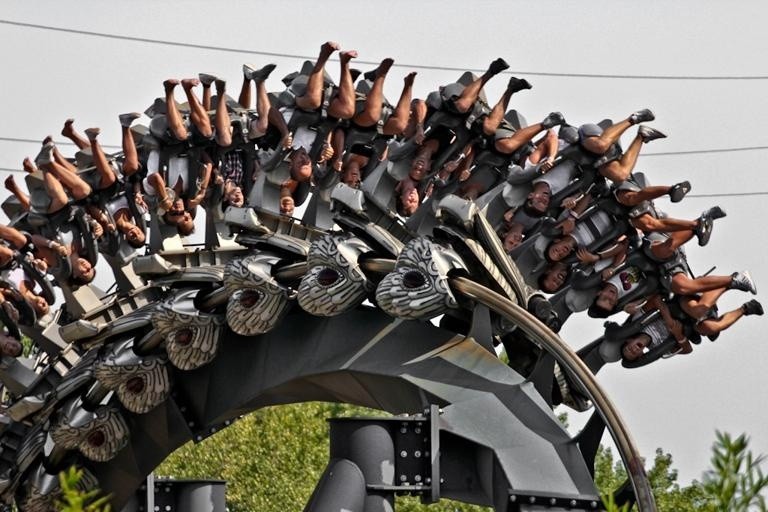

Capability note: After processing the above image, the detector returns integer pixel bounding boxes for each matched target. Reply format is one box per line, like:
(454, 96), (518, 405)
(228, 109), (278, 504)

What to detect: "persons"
(0, 41), (765, 360)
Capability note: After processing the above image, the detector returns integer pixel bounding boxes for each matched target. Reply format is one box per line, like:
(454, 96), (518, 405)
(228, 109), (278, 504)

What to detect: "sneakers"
(35, 140), (54, 169)
(668, 180), (692, 203)
(119, 110), (140, 129)
(542, 112), (566, 128)
(199, 71), (215, 87)
(630, 107), (655, 124)
(702, 206), (726, 220)
(507, 75), (533, 93)
(742, 298), (764, 316)
(490, 58), (508, 73)
(84, 127), (101, 142)
(638, 125), (668, 144)
(694, 216), (713, 247)
(726, 270), (758, 293)
(216, 78), (226, 92)
(240, 61), (275, 82)
